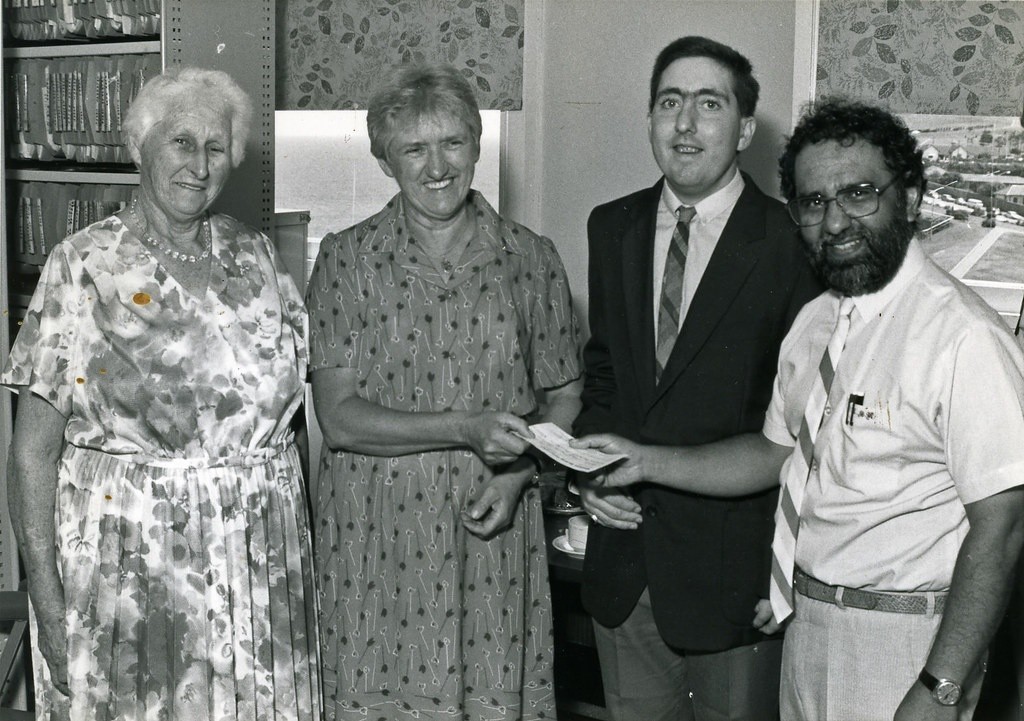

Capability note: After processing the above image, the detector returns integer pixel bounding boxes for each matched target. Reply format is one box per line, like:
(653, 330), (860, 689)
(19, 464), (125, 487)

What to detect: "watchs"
(918, 666), (966, 707)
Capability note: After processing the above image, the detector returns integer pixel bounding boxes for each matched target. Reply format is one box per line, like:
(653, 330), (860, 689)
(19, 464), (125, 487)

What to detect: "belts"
(791, 569), (948, 618)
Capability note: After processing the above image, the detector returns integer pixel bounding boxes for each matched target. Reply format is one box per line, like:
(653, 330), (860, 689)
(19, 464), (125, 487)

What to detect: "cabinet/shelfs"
(4, 0), (281, 334)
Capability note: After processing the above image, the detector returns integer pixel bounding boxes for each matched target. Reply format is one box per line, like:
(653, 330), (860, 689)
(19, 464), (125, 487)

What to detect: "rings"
(591, 514), (598, 522)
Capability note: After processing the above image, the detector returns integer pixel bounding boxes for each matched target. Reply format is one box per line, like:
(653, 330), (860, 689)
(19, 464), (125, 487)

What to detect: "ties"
(654, 206), (697, 388)
(769, 296), (860, 625)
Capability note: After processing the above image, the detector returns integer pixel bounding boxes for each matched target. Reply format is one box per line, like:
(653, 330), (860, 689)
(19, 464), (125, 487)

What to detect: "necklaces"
(413, 216), (472, 274)
(129, 192), (212, 264)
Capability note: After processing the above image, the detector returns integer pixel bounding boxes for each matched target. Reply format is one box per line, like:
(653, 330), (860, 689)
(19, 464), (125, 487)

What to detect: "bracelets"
(519, 452), (542, 486)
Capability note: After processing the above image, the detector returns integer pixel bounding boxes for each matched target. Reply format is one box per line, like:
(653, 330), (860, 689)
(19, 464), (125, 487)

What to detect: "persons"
(302, 63), (586, 721)
(0, 65), (323, 721)
(567, 93), (1024, 721)
(570, 34), (828, 721)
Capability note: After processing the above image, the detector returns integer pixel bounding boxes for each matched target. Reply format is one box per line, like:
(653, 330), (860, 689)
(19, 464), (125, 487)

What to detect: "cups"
(567, 516), (590, 551)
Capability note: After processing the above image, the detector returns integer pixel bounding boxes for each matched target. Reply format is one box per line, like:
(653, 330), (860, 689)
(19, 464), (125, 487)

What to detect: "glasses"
(784, 167), (905, 228)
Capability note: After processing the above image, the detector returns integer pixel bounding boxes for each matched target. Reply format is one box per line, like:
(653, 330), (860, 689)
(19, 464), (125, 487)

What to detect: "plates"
(553, 535), (586, 559)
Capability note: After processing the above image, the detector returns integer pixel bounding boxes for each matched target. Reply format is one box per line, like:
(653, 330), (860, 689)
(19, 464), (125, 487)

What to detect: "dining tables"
(531, 467), (587, 590)
